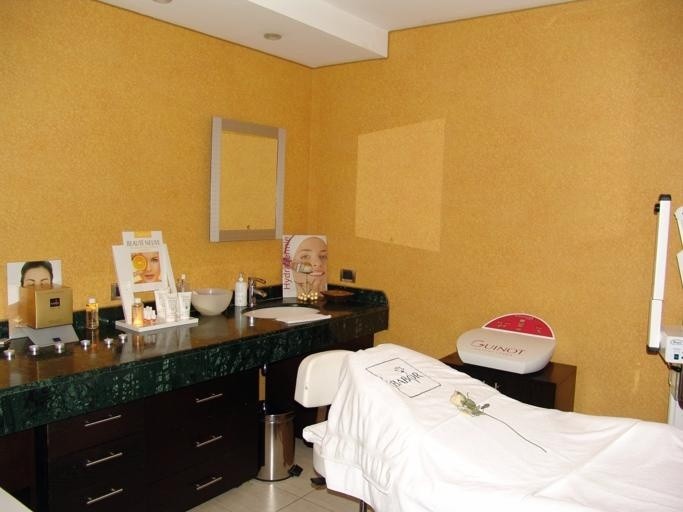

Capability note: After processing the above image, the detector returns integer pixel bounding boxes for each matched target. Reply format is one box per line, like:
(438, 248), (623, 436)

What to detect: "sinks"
(240, 302), (332, 327)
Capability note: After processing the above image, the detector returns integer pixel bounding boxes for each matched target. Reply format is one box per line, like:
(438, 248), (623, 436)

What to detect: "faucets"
(248, 276), (268, 307)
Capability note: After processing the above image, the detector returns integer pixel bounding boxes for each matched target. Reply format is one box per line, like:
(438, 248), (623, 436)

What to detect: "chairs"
(294, 349), (356, 486)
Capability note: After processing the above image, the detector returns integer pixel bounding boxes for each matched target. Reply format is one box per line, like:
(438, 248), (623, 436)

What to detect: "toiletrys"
(235, 271), (247, 306)
(154, 274), (192, 321)
(86, 299), (99, 329)
(132, 298), (144, 327)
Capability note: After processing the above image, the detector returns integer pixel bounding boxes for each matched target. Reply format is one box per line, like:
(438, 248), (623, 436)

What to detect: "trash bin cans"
(253, 403), (303, 481)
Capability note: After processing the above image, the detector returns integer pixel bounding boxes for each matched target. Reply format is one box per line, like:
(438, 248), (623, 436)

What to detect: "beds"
(312, 342), (682, 512)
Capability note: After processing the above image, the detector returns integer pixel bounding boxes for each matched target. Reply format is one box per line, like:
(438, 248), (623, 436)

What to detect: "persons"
(21, 261), (53, 287)
(131, 252), (162, 284)
(284, 234), (328, 297)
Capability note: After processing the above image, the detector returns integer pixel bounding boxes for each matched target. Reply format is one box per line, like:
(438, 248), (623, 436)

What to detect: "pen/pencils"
(176, 273), (186, 292)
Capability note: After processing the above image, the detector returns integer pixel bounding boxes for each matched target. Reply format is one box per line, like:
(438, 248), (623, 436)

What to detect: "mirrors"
(209, 114), (286, 244)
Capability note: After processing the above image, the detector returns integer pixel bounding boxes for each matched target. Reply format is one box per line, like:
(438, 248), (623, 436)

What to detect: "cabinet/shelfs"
(0, 332), (375, 512)
(440, 349), (577, 412)
